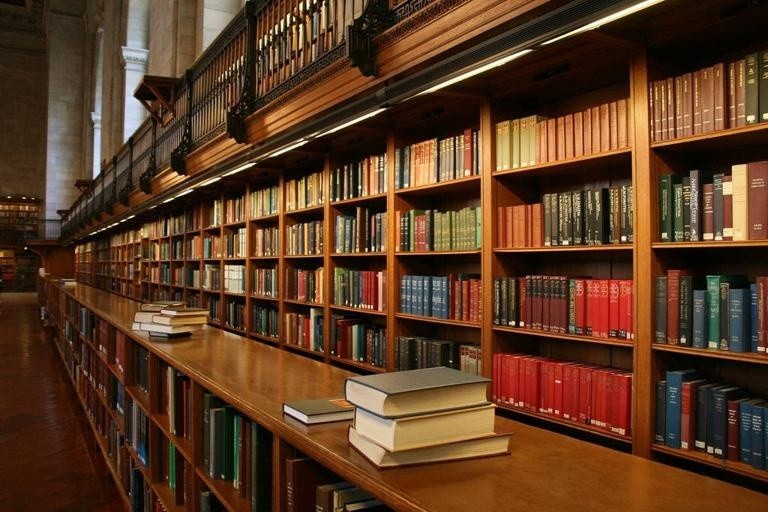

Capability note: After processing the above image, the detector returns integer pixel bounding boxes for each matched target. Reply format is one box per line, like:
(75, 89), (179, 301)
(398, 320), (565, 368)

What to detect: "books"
(2, 194), (40, 289)
(37, 2), (389, 512)
(345, 47), (768, 472)
